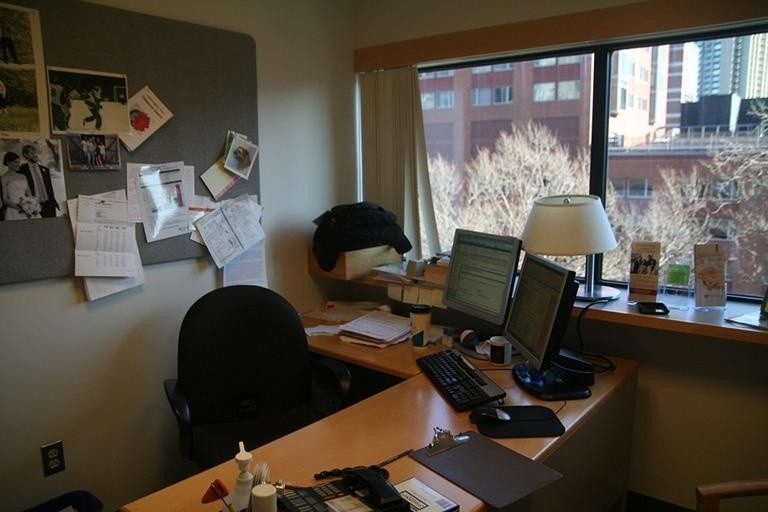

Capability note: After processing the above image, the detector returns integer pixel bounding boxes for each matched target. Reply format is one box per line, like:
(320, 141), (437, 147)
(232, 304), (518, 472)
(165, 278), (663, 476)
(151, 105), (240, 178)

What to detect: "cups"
(482, 336), (512, 366)
(408, 303), (431, 350)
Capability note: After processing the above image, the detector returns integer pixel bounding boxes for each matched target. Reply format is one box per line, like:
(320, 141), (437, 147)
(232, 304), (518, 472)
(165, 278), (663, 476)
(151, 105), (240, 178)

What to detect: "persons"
(0, 78), (8, 116)
(49, 83), (75, 130)
(80, 136), (106, 167)
(1, 151), (42, 219)
(82, 87), (104, 129)
(630, 254), (657, 274)
(17, 145), (60, 217)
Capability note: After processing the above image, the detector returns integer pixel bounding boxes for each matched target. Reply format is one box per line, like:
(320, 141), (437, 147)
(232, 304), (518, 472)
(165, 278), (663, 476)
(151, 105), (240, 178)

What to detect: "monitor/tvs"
(442, 229), (523, 359)
(503, 253), (591, 400)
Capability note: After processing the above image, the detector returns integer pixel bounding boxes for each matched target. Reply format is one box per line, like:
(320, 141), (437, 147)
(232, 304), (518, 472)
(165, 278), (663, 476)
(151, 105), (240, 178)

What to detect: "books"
(337, 309), (413, 350)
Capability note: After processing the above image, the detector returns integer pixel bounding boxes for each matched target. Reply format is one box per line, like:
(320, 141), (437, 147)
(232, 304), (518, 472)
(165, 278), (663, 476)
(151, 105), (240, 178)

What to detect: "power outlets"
(41, 440), (66, 477)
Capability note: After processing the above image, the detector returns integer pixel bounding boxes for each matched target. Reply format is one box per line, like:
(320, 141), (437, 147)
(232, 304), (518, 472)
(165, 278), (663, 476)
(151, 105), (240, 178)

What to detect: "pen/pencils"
(378, 448), (414, 467)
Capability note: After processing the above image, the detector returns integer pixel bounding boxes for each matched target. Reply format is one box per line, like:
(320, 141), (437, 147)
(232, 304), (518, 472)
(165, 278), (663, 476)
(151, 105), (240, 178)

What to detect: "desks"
(296, 292), (465, 378)
(115, 376), (637, 512)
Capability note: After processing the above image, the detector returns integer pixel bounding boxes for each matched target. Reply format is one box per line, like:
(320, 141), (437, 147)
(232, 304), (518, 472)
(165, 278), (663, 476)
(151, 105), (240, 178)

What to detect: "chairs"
(164, 284), (353, 475)
(695, 477), (768, 512)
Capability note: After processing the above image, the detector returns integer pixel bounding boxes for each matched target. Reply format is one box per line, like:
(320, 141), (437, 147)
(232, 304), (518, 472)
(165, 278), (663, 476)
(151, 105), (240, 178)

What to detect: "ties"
(32, 164), (46, 204)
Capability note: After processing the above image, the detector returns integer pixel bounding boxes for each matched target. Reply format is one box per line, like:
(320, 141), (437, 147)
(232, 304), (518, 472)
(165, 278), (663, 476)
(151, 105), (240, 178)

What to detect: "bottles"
(250, 483), (278, 511)
(275, 479), (286, 499)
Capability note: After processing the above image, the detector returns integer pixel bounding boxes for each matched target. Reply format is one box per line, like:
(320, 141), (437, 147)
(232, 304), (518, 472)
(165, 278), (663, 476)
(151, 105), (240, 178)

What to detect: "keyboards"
(416, 348), (507, 412)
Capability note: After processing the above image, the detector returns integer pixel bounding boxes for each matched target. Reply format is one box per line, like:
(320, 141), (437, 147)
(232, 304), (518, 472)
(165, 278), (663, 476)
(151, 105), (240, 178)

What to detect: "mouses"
(480, 407), (511, 423)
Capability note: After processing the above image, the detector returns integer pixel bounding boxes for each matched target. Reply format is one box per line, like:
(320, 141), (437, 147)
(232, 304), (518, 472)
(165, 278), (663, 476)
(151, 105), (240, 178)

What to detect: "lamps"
(518, 192), (620, 302)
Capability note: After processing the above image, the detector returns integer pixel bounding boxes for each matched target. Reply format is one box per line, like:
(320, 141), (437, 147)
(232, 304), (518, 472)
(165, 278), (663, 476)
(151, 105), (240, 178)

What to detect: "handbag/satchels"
(313, 202), (397, 251)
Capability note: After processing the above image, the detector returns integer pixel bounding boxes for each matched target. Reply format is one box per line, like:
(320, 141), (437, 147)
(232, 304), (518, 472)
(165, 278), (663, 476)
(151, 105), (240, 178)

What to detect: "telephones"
(277, 468), (410, 512)
